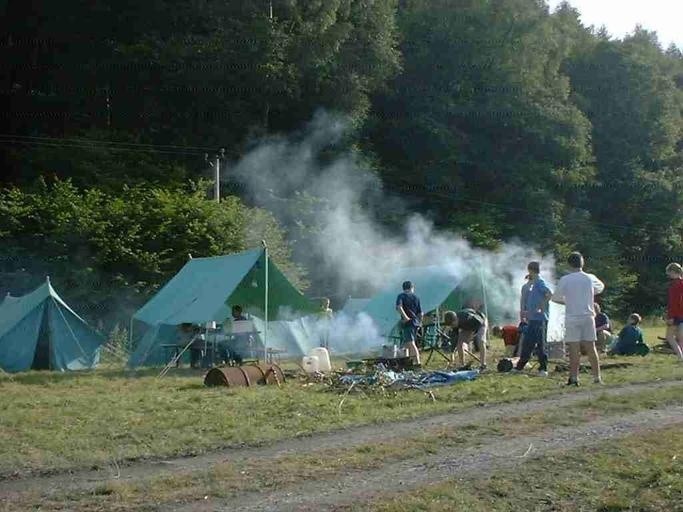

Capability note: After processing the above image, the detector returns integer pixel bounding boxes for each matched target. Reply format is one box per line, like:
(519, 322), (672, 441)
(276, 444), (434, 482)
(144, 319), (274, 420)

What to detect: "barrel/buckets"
(382, 344), (397, 360)
(303, 355), (320, 373)
(204, 363), (287, 387)
(310, 346), (331, 373)
(396, 348), (410, 358)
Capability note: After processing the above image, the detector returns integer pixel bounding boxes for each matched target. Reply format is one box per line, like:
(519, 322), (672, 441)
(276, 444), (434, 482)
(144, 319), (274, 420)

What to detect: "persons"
(442, 307), (488, 371)
(177, 322), (201, 368)
(395, 281), (424, 366)
(489, 325), (520, 352)
(550, 250), (607, 385)
(662, 261), (682, 358)
(512, 260), (553, 376)
(588, 302), (612, 353)
(604, 311), (649, 356)
(317, 297), (334, 347)
(218, 305), (251, 366)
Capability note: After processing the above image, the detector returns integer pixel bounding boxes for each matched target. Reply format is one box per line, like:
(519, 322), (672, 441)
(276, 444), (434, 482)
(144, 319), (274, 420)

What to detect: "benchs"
(246, 345), (287, 365)
(159, 343), (185, 368)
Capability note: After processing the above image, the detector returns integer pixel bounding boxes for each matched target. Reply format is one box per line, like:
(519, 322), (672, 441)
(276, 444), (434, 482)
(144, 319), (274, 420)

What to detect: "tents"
(335, 294), (368, 319)
(0, 280), (105, 375)
(127, 247), (322, 366)
(363, 256), (520, 353)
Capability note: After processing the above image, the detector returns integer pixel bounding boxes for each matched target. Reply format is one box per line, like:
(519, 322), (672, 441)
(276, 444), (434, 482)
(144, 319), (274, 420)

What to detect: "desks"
(194, 326), (260, 367)
